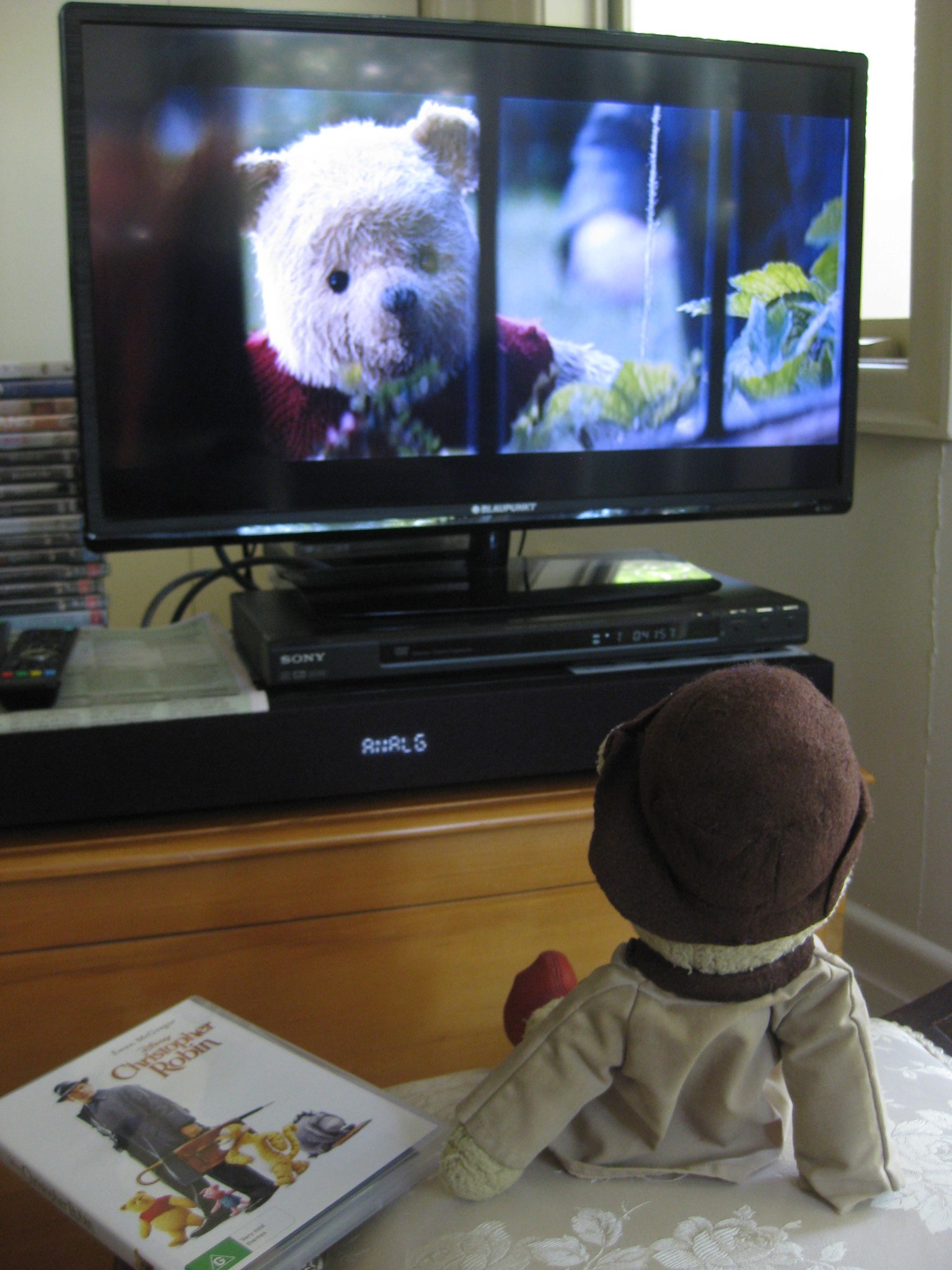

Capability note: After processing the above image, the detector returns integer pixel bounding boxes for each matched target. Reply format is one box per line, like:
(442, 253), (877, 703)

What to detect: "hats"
(53, 1076), (89, 1102)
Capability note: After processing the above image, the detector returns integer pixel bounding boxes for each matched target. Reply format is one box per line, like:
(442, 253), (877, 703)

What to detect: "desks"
(0, 763), (874, 1270)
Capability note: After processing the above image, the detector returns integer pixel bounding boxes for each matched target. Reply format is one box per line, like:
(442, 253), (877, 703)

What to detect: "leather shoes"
(191, 1211), (230, 1237)
(245, 1183), (278, 1212)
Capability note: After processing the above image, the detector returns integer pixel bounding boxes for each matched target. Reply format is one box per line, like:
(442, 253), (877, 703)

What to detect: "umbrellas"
(136, 1099), (277, 1184)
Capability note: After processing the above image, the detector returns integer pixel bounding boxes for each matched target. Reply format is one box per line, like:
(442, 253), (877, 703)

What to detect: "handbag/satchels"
(173, 1117), (256, 1171)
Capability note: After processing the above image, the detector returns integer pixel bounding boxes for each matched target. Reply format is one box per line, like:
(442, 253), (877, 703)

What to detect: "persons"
(54, 1076), (279, 1237)
(552, 103), (845, 299)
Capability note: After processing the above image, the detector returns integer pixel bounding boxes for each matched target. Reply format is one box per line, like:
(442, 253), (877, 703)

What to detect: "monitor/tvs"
(57, 1), (871, 624)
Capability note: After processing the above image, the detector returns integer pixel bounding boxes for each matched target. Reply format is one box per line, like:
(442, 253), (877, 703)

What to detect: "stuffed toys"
(444, 664), (903, 1215)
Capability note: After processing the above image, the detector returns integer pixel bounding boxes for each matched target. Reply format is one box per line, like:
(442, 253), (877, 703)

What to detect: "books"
(0, 361), (109, 632)
(0, 996), (446, 1270)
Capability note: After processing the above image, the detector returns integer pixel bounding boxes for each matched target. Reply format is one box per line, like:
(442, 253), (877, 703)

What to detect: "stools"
(314, 1018), (952, 1270)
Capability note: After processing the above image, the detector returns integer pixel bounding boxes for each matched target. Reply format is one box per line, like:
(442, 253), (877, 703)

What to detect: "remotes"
(1, 625), (80, 712)
